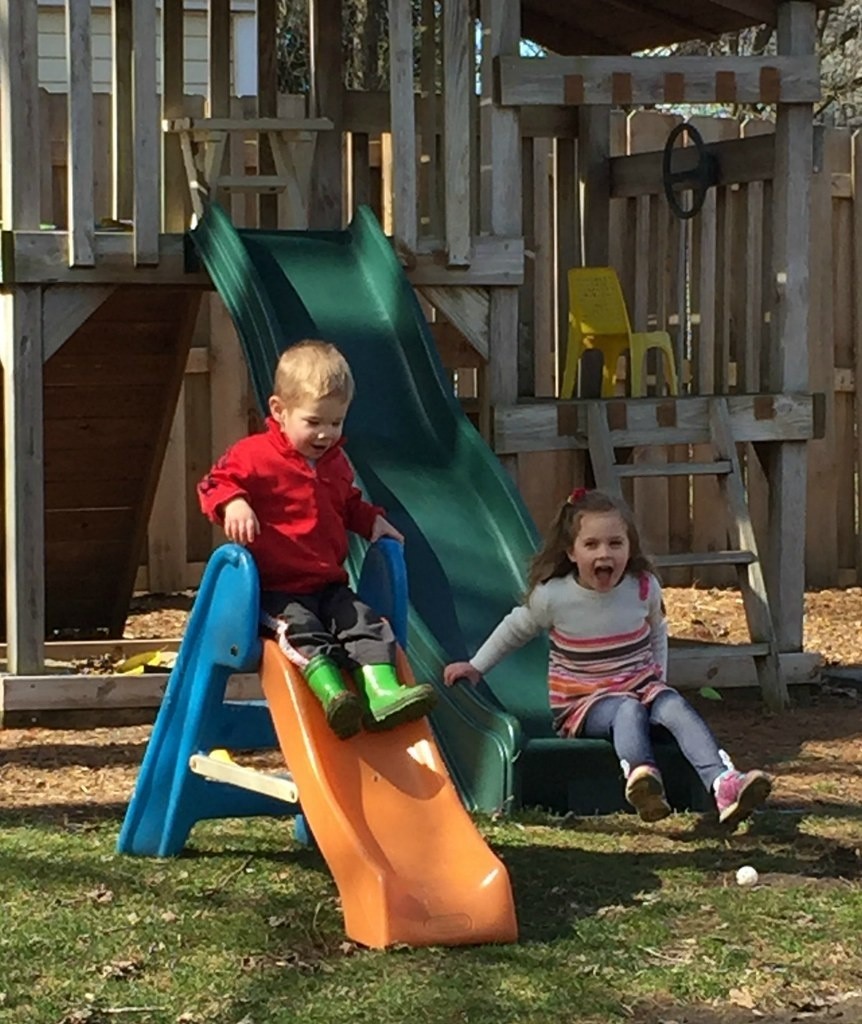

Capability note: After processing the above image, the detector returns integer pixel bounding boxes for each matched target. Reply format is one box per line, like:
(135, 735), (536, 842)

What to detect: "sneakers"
(715, 771), (772, 829)
(623, 764), (671, 823)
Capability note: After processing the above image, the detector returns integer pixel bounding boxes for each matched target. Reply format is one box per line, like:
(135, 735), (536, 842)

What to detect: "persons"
(195, 339), (435, 742)
(442, 489), (772, 828)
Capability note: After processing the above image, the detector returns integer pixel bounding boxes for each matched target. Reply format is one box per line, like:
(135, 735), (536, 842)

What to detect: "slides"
(261, 617), (519, 951)
(183, 199), (705, 816)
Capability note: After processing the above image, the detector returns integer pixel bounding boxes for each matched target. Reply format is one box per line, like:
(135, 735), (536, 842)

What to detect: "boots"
(350, 664), (439, 733)
(299, 656), (364, 742)
